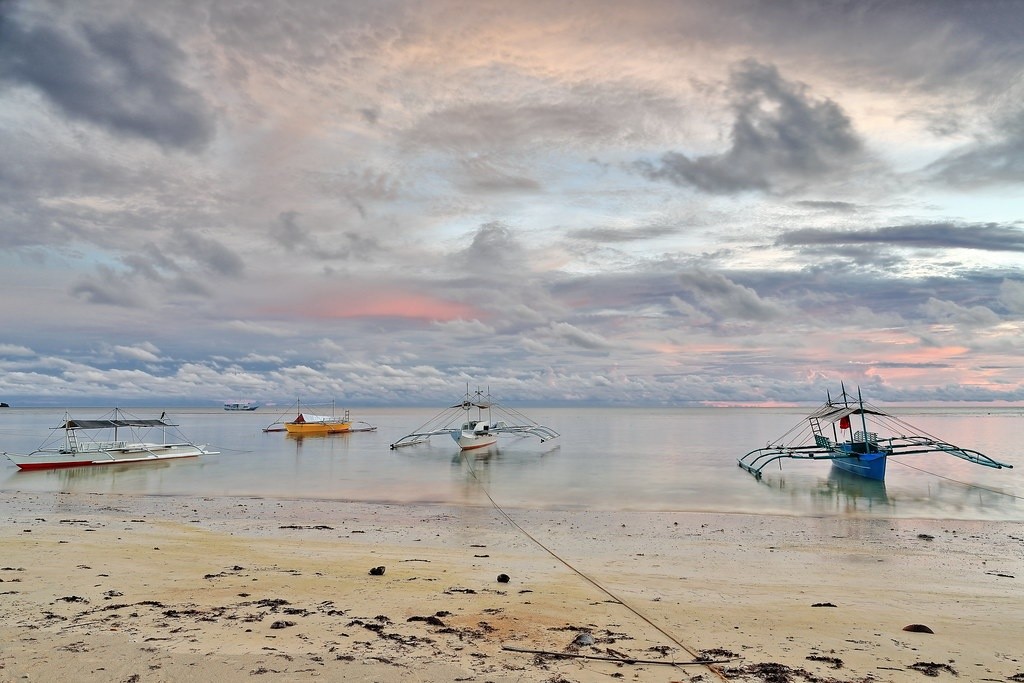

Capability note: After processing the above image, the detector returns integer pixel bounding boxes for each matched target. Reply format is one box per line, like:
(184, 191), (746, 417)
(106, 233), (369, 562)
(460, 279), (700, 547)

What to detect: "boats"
(261, 397), (378, 435)
(224, 399), (261, 411)
(392, 382), (561, 449)
(735, 379), (1015, 481)
(1, 406), (220, 470)
